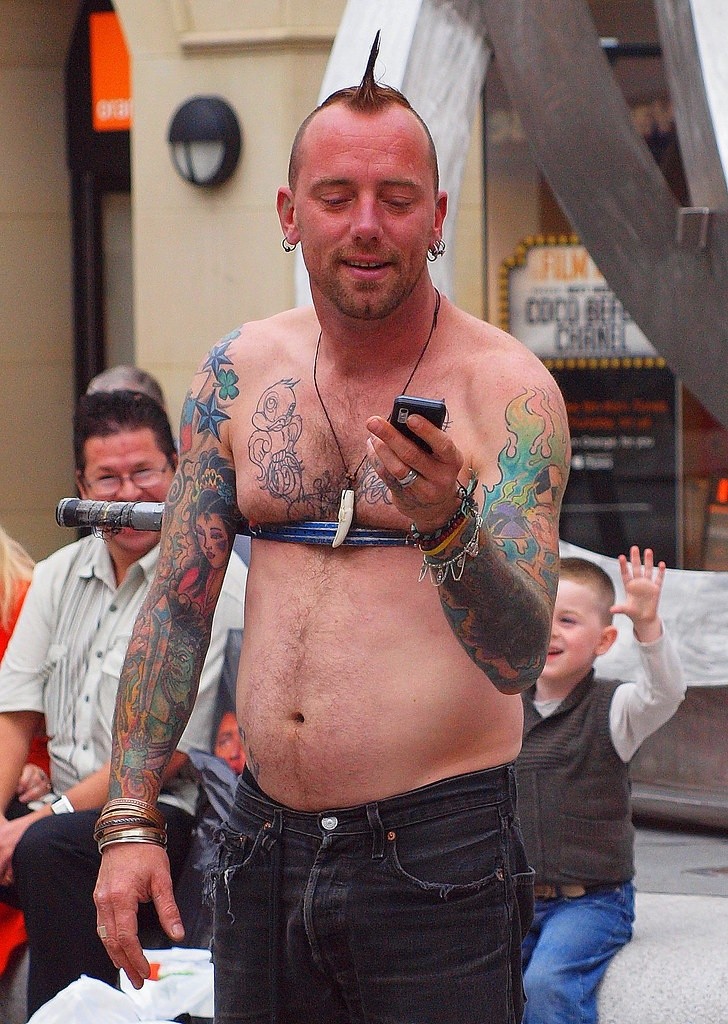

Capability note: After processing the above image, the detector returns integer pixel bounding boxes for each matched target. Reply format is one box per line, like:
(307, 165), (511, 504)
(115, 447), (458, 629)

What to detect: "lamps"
(165, 94), (240, 192)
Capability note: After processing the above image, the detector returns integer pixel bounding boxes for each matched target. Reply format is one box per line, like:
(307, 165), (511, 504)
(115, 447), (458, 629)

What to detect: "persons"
(0, 519), (66, 1019)
(84, 362), (259, 573)
(87, 21), (576, 1021)
(496, 546), (689, 1024)
(0, 385), (257, 1024)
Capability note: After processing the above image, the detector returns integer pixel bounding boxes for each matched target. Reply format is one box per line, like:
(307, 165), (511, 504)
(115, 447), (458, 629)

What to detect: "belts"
(533, 883), (588, 899)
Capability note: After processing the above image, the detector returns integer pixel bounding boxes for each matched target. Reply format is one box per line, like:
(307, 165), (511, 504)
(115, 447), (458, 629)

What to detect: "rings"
(399, 465), (420, 488)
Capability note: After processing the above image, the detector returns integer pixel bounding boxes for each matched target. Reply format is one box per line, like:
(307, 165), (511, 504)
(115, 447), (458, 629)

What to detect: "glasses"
(82, 455), (171, 496)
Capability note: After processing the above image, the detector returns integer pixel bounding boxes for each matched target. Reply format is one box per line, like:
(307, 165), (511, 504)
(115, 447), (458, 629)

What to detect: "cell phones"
(391, 395), (446, 453)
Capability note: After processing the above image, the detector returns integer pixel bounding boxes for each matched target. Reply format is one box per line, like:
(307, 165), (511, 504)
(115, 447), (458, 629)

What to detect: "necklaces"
(310, 275), (446, 552)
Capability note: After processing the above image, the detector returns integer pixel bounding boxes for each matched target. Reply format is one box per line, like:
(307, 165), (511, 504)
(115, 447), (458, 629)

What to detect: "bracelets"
(404, 480), (485, 587)
(93, 792), (171, 856)
